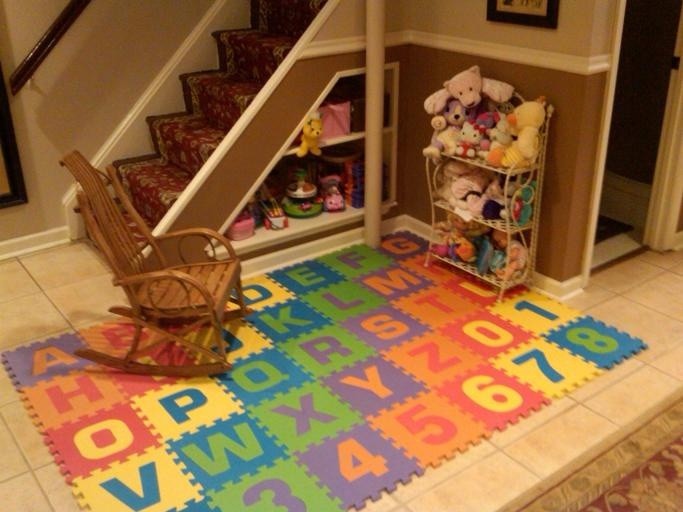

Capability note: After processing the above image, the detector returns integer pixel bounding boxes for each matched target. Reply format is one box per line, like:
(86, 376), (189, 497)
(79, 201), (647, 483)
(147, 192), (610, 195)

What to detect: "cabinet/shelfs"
(423, 91), (552, 303)
(207, 61), (402, 258)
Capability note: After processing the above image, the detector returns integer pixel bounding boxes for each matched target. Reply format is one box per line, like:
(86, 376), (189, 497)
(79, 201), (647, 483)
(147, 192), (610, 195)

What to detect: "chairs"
(59, 147), (251, 376)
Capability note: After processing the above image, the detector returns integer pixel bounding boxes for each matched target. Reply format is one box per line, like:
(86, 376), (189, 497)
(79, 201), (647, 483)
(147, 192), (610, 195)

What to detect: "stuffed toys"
(296, 110), (323, 156)
(423, 64), (546, 282)
(242, 182), (289, 228)
(314, 171), (346, 211)
(277, 166), (324, 220)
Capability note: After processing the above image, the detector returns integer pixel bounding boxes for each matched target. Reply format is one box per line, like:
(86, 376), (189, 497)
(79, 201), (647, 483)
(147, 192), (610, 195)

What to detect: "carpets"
(490, 389), (680, 511)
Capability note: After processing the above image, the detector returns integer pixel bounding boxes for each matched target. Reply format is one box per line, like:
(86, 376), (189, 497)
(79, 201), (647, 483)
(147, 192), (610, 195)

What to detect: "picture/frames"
(485, 0), (560, 30)
